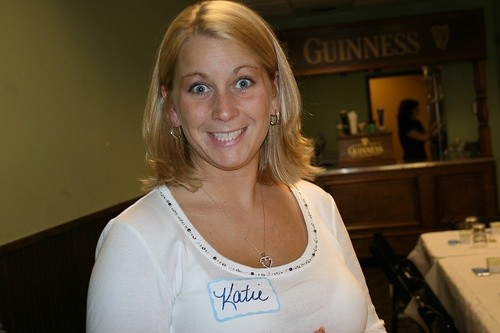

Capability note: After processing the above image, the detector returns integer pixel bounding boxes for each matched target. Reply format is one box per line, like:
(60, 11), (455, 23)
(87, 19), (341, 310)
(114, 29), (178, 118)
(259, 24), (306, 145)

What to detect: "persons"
(88, 1), (386, 332)
(397, 99), (437, 162)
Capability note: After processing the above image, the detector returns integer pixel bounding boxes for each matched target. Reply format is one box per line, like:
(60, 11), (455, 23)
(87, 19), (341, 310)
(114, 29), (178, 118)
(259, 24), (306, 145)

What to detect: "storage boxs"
(337, 131), (395, 165)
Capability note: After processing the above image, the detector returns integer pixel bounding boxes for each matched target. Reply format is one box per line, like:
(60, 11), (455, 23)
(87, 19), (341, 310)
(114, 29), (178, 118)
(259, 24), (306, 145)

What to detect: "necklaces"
(198, 183), (274, 270)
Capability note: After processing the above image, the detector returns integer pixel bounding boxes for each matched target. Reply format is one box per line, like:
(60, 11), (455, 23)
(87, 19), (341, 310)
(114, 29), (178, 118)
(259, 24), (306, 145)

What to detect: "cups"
(473, 223), (486, 242)
(465, 217), (477, 238)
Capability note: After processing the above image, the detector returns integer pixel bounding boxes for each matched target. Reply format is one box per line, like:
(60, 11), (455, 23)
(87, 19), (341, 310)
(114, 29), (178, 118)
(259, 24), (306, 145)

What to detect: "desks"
(421, 227), (500, 333)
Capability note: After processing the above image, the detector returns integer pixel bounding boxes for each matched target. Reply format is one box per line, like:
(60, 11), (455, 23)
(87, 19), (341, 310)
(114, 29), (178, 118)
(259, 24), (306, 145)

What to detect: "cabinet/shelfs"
(300, 154), (500, 259)
